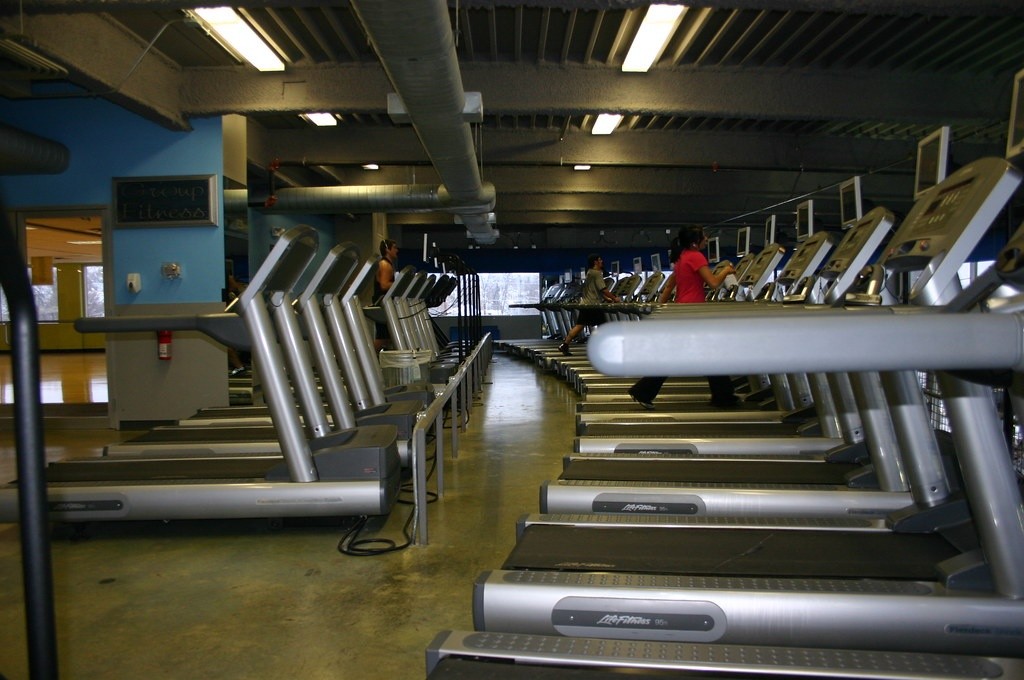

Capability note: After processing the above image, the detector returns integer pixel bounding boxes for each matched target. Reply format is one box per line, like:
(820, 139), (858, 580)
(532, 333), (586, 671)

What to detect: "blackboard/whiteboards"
(111, 175), (217, 228)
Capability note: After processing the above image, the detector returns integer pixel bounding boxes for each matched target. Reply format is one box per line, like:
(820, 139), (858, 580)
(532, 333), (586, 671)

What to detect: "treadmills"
(419, 68), (1024, 680)
(0, 221), (461, 524)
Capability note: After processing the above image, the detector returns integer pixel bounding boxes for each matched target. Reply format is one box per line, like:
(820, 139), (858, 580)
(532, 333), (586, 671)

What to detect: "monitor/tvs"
(839, 176), (862, 229)
(797, 200), (813, 242)
(708, 237), (719, 263)
(913, 126), (949, 202)
(765, 214), (776, 246)
(423, 235), (446, 276)
(736, 226), (751, 257)
(564, 248), (675, 284)
(1006, 69), (1024, 162)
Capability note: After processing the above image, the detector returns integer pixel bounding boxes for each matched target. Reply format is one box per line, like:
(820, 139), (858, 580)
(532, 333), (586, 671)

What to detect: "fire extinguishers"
(156, 330), (173, 361)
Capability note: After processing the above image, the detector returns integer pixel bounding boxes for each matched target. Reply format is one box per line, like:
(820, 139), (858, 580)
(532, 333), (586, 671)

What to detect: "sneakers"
(723, 397), (746, 407)
(628, 388), (657, 410)
(558, 342), (573, 357)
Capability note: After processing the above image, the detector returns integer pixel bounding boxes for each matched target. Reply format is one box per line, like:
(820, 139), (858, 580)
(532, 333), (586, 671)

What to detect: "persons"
(558, 255), (620, 356)
(228, 270), (247, 378)
(374, 239), (398, 349)
(628, 225), (741, 409)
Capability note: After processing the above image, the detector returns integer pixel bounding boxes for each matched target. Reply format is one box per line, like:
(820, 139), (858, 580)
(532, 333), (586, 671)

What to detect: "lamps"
(179, 4), (337, 126)
(574, 1), (691, 171)
